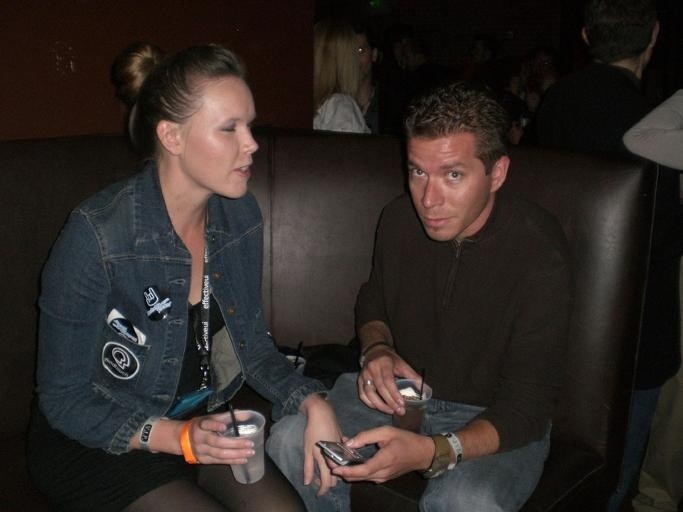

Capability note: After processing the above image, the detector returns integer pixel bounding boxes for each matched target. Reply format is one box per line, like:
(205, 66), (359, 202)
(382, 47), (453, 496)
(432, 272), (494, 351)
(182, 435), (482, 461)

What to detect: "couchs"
(1, 126), (660, 512)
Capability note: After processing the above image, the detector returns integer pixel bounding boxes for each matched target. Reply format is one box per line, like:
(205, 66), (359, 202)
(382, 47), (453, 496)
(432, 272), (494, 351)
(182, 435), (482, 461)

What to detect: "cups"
(282, 354), (309, 373)
(389, 379), (433, 431)
(216, 411), (272, 483)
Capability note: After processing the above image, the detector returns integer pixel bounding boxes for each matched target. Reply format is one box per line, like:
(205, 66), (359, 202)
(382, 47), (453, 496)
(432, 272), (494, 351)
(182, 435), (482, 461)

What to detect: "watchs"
(419, 433), (451, 480)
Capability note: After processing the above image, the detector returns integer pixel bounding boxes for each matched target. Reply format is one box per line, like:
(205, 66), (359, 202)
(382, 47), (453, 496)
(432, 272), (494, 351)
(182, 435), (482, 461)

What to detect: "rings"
(361, 378), (373, 389)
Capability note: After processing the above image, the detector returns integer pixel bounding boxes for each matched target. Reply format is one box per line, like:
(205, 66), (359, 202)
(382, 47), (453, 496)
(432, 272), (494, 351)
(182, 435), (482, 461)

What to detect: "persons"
(440, 431), (463, 472)
(24, 41), (343, 512)
(264, 82), (577, 512)
(315, 20), (376, 135)
(528, 0), (680, 511)
(373, 31), (561, 144)
(623, 88), (681, 510)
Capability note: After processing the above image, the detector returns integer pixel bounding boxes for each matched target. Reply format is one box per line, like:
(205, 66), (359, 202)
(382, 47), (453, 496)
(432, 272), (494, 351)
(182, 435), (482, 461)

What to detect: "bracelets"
(138, 413), (171, 456)
(178, 416), (199, 465)
(358, 341), (395, 368)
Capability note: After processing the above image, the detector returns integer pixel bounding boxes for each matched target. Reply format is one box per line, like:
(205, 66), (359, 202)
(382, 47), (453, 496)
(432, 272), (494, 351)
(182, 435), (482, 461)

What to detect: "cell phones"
(315, 438), (366, 469)
